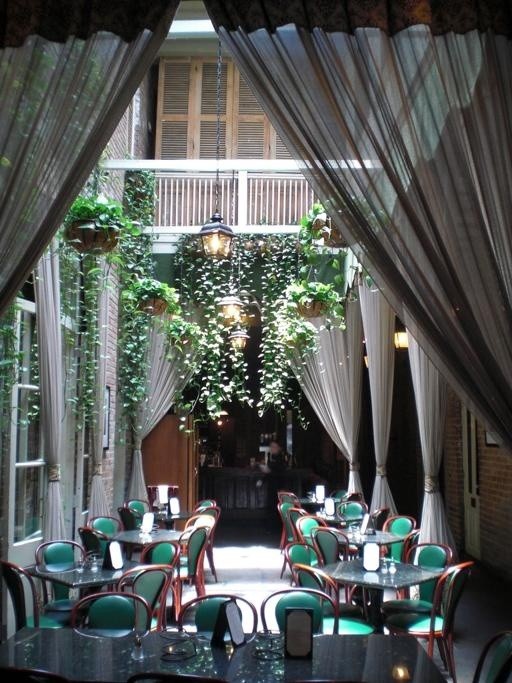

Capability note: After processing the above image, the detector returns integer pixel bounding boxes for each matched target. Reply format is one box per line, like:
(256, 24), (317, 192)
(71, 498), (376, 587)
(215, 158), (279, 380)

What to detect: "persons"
(258, 441), (288, 534)
(303, 464), (330, 513)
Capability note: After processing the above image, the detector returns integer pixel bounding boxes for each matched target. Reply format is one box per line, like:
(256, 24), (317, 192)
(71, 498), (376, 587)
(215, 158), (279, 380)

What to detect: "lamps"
(197, 30), (237, 268)
(227, 252), (251, 352)
(393, 313), (408, 354)
(214, 169), (245, 328)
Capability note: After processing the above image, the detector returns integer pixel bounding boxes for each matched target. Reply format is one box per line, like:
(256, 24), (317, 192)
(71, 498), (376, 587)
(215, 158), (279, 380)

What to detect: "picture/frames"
(99, 380), (111, 451)
(483, 422), (501, 448)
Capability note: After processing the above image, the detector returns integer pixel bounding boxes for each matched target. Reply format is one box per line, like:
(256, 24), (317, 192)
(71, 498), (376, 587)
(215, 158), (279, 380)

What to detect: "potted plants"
(294, 192), (399, 270)
(54, 187), (144, 259)
(274, 278), (350, 324)
(115, 268), (182, 321)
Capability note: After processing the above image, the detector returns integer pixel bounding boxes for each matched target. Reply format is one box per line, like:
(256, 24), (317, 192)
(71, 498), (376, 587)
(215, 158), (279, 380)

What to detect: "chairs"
(277, 488), (475, 682)
(177, 595), (258, 635)
(259, 587), (340, 636)
(1, 484), (220, 630)
(472, 632), (511, 681)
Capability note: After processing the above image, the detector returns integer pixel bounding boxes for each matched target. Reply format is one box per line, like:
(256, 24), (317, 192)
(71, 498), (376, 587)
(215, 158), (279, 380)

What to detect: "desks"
(0, 626), (448, 682)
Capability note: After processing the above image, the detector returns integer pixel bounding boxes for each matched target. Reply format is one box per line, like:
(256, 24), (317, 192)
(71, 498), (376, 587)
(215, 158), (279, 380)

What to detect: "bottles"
(77, 554), (99, 574)
(381, 555), (396, 574)
(130, 637), (144, 661)
